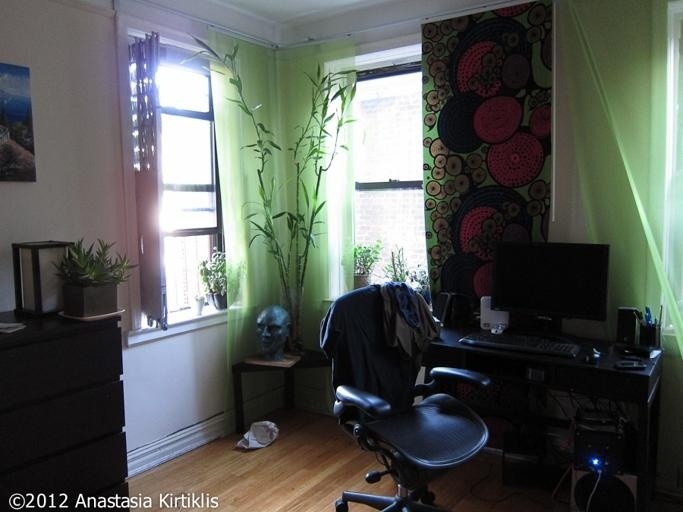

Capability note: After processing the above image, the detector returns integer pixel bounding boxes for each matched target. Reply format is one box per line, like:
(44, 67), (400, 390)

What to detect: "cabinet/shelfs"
(2, 308), (128, 512)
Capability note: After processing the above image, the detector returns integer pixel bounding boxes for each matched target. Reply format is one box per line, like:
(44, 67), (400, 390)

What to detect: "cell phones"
(613, 357), (649, 370)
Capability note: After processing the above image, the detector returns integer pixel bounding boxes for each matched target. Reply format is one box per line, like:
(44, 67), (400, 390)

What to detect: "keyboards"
(458, 329), (577, 361)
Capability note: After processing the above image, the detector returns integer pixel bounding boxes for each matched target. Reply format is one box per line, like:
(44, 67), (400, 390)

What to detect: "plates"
(57, 309), (126, 321)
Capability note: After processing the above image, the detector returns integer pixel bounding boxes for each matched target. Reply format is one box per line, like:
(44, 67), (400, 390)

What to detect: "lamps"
(12, 239), (75, 316)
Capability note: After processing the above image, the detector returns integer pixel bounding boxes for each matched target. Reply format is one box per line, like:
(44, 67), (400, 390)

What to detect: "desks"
(419, 327), (664, 512)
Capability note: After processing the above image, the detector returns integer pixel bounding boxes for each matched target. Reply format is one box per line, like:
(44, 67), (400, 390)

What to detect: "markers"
(644, 305), (653, 327)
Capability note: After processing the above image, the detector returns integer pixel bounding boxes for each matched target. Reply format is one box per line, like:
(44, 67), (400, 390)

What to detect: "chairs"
(319, 281), (491, 512)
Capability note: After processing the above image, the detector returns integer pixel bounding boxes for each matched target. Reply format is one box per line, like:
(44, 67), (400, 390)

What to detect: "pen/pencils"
(658, 305), (663, 325)
(633, 311), (643, 327)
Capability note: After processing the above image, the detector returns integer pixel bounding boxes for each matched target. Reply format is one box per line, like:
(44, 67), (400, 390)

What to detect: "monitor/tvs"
(490, 239), (610, 339)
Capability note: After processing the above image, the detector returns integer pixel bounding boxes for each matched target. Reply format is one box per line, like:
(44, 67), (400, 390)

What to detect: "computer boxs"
(567, 404), (631, 475)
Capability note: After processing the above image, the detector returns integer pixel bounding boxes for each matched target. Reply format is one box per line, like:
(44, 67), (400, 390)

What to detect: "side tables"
(232, 349), (332, 434)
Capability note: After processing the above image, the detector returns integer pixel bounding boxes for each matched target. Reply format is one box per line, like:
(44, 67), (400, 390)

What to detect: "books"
(244, 356), (302, 369)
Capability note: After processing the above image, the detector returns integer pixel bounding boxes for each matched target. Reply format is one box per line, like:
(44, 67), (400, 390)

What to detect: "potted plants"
(179, 29), (356, 355)
(52, 237), (139, 323)
(342, 240), (385, 290)
(198, 248), (242, 309)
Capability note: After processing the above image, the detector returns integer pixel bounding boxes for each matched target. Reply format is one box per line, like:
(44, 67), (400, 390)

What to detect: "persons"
(255, 305), (292, 362)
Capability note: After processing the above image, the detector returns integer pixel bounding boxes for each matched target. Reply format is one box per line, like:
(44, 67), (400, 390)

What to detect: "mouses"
(572, 346), (602, 366)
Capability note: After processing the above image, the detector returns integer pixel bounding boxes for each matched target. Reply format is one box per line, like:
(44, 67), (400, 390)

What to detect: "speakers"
(448, 291), (474, 331)
(615, 306), (643, 351)
